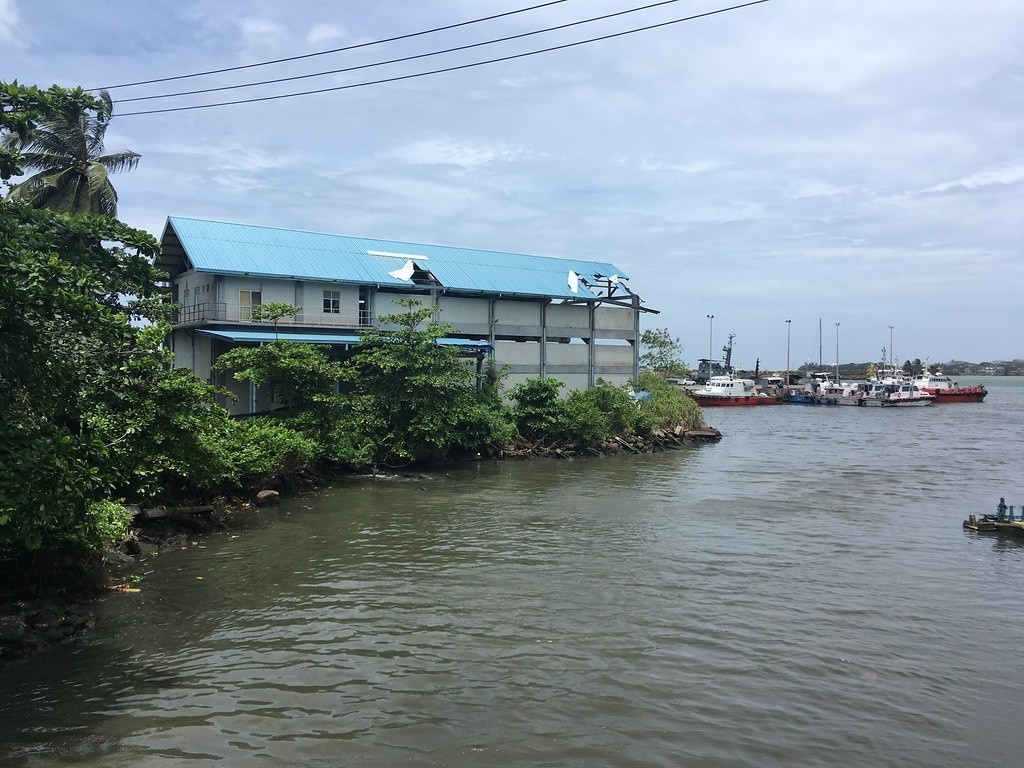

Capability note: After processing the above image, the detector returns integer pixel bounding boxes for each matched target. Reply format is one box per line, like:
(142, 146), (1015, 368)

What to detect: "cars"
(677, 378), (696, 385)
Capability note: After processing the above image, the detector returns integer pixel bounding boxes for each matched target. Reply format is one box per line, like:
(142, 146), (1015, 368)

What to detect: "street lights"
(785, 319), (793, 387)
(888, 325), (896, 371)
(707, 315), (715, 385)
(834, 321), (839, 381)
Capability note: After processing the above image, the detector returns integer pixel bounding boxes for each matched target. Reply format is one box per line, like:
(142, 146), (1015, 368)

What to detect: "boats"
(690, 378), (785, 406)
(781, 367), (988, 406)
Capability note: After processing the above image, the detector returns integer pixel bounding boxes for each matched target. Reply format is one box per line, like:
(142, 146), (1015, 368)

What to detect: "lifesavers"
(745, 397), (749, 401)
(751, 393), (756, 397)
(735, 398), (739, 402)
(898, 393), (901, 397)
(936, 388), (940, 395)
(776, 396), (781, 401)
(885, 399), (937, 404)
(695, 395), (732, 402)
(816, 399), (822, 404)
